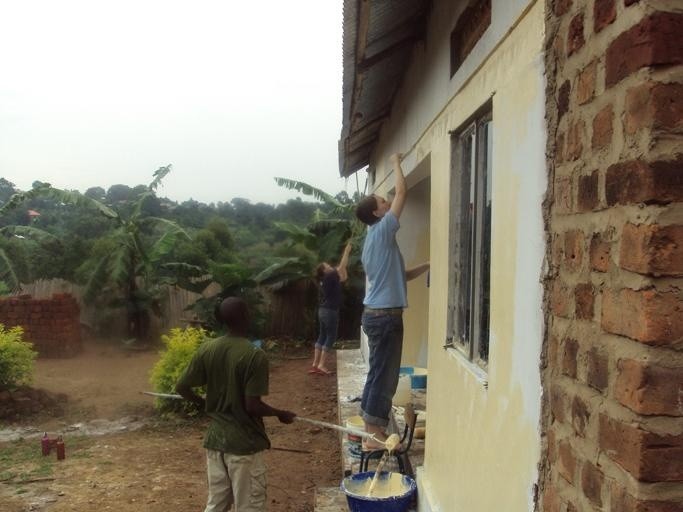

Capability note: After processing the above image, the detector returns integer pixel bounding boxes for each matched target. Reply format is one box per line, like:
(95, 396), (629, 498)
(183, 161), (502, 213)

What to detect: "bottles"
(41, 430), (50, 455)
(55, 436), (65, 461)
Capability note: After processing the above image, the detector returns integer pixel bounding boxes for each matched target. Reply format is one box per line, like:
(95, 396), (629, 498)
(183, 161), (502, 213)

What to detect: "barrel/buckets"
(346, 416), (364, 447)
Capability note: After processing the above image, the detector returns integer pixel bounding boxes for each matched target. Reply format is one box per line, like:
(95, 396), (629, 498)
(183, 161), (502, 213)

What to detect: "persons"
(173, 296), (299, 512)
(307, 242), (352, 375)
(358, 153), (429, 452)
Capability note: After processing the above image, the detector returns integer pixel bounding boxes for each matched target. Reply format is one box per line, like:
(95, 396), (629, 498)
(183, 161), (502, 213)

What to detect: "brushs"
(138, 391), (400, 451)
(349, 167), (376, 245)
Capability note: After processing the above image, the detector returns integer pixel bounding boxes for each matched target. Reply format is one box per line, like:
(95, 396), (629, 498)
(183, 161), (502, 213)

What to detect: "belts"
(363, 306), (402, 315)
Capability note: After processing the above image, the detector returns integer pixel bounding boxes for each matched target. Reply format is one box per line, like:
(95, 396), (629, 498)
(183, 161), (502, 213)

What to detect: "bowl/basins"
(396, 367), (427, 388)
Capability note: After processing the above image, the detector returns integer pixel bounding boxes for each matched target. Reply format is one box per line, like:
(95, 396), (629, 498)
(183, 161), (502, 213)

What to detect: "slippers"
(308, 365), (331, 374)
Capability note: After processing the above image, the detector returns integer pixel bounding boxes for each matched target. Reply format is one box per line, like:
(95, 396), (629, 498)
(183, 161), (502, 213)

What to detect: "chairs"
(358, 405), (418, 475)
